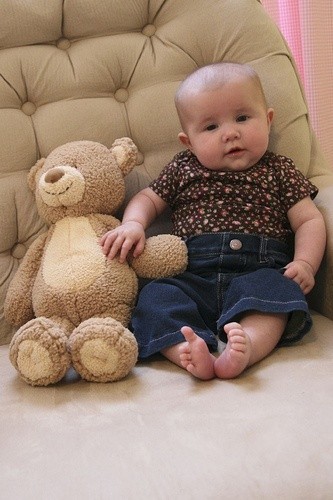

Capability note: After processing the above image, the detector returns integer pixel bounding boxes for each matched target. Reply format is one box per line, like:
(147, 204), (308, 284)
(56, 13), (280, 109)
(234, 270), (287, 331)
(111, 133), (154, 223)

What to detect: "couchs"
(0, 1), (333, 500)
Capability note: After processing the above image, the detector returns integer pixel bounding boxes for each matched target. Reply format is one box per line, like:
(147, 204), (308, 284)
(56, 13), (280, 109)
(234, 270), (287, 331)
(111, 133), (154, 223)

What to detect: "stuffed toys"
(3, 137), (189, 386)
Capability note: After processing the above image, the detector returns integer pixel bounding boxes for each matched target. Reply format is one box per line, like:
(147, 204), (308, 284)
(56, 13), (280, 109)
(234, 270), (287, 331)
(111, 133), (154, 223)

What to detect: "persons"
(101, 62), (326, 381)
